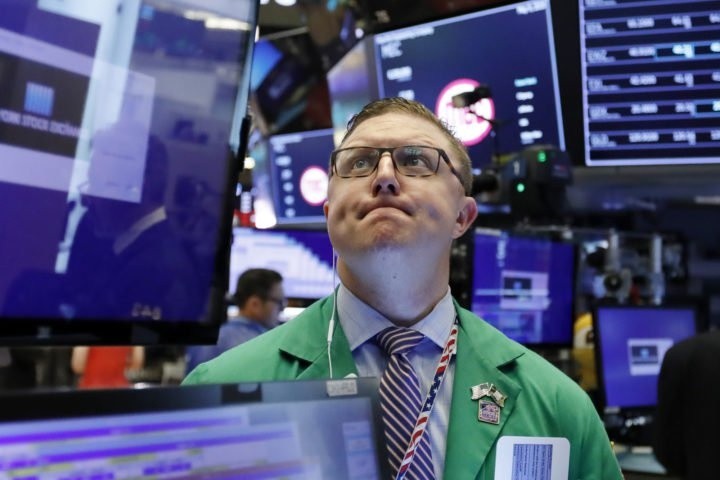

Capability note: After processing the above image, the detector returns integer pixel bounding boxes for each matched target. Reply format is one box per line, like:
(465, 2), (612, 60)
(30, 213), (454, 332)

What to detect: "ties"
(373, 326), (443, 480)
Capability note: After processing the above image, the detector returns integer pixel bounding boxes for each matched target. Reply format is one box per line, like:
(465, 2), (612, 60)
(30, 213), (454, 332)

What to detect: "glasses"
(269, 296), (289, 308)
(328, 145), (466, 190)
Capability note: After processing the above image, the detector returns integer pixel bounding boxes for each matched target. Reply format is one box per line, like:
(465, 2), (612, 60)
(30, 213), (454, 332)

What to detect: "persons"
(71, 346), (145, 390)
(647, 289), (719, 480)
(180, 269), (288, 379)
(180, 95), (621, 480)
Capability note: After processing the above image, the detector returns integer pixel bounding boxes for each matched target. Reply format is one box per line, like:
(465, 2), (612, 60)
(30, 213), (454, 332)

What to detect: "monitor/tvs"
(0, 1), (263, 348)
(590, 303), (701, 421)
(266, 0), (720, 230)
(469, 223), (579, 349)
(1, 377), (393, 479)
